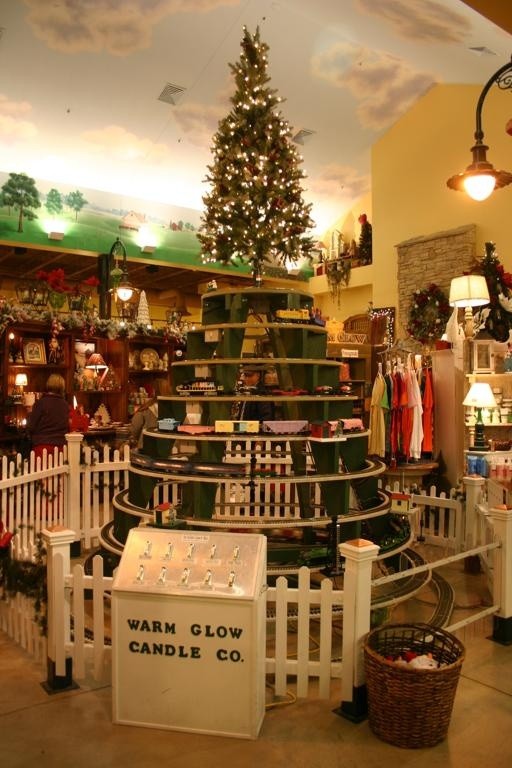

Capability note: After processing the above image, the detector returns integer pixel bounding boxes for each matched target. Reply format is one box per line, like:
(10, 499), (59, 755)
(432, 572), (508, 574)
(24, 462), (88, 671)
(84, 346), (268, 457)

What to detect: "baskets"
(362, 620), (466, 750)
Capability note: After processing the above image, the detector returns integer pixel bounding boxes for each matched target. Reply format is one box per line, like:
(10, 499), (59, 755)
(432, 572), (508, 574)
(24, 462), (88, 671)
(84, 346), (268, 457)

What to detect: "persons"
(25, 373), (71, 521)
(127, 378), (170, 453)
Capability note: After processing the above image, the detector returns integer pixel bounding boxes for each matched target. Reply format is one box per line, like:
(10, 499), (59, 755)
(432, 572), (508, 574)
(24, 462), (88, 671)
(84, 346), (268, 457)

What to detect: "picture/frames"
(19, 336), (48, 366)
(468, 336), (496, 376)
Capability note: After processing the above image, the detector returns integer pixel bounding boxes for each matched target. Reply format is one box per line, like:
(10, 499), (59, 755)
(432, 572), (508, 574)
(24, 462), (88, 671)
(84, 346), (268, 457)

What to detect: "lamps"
(447, 273), (490, 345)
(458, 381), (498, 452)
(442, 61), (512, 204)
(46, 230), (66, 242)
(287, 266), (301, 276)
(15, 371), (27, 393)
(140, 245), (158, 255)
(95, 238), (134, 306)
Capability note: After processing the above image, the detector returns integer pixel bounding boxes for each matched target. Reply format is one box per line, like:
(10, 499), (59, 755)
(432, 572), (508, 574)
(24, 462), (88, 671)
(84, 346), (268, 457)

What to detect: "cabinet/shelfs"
(1, 314), (73, 455)
(320, 337), (375, 434)
(96, 332), (173, 428)
(474, 371), (512, 426)
(79, 285), (452, 653)
(66, 326), (127, 455)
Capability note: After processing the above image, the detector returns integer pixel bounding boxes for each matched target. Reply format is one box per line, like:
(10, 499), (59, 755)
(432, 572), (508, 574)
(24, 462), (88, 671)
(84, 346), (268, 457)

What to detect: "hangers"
(370, 338), (436, 381)
